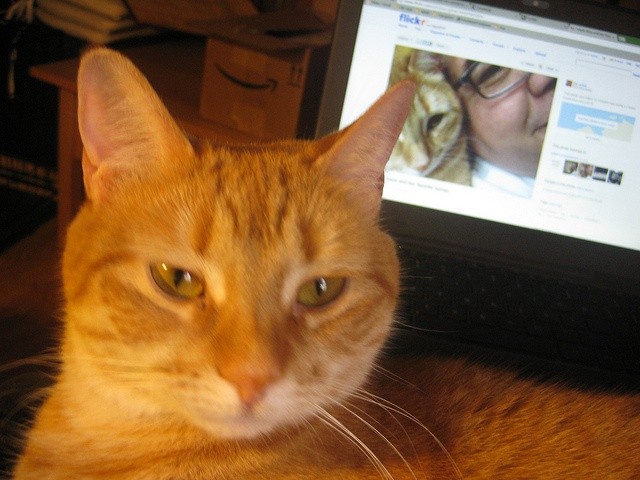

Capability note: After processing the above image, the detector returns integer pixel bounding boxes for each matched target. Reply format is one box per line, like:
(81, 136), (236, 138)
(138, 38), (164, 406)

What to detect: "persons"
(441, 52), (559, 181)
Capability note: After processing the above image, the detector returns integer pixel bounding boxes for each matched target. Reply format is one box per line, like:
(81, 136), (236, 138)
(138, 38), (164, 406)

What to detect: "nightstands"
(28, 27), (308, 251)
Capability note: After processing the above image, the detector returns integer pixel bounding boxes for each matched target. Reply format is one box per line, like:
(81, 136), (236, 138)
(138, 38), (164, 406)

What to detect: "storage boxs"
(198, 23), (330, 140)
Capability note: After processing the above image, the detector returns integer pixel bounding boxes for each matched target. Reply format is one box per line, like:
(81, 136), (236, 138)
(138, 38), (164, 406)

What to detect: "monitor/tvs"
(312, 2), (638, 392)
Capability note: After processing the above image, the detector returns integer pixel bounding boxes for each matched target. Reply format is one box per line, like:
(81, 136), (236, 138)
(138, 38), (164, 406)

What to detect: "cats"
(382, 44), (475, 186)
(1, 45), (640, 480)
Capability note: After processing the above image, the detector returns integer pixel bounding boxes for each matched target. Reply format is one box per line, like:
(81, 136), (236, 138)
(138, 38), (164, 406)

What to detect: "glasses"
(452, 61), (529, 100)
